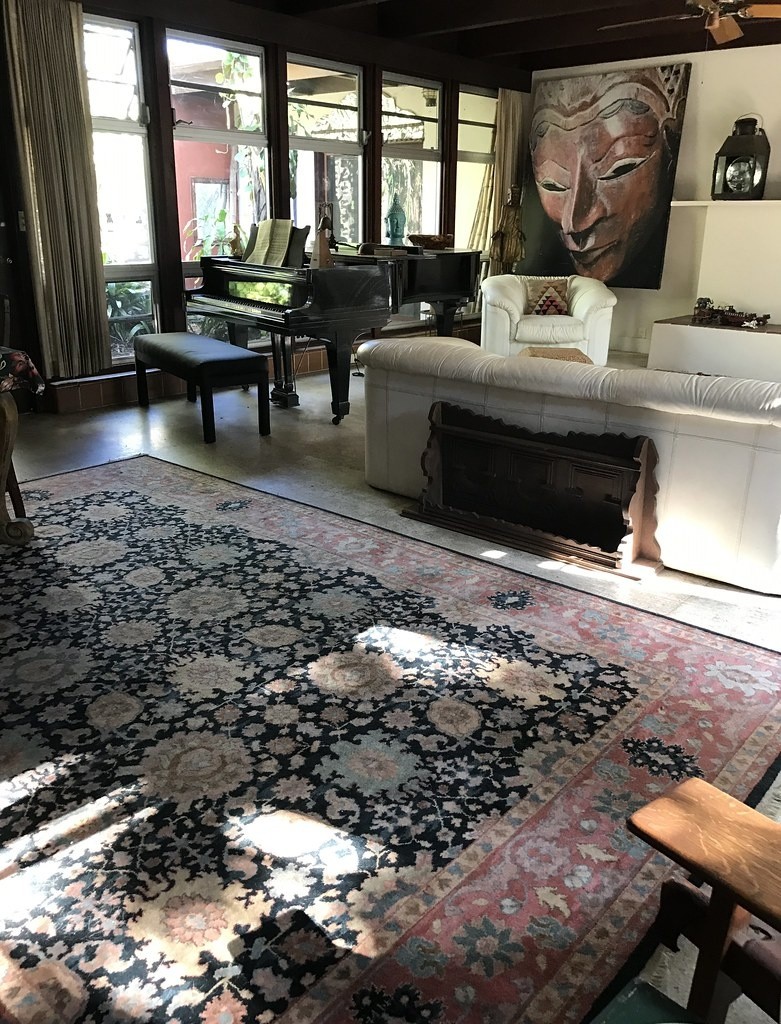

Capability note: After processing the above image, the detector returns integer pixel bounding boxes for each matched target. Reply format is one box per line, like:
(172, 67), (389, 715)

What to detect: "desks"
(305, 249), (483, 336)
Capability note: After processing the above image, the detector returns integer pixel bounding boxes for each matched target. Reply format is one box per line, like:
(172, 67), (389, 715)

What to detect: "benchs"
(132, 331), (271, 445)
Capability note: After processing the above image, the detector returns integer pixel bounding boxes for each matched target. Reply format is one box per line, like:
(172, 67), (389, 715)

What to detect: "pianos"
(181, 249), (480, 425)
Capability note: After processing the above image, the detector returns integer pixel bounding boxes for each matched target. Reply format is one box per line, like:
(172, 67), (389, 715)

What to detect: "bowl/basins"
(408, 234), (454, 250)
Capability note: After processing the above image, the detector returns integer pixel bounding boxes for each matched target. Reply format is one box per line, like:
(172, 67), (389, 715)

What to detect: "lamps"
(421, 87), (437, 109)
(704, 12), (720, 31)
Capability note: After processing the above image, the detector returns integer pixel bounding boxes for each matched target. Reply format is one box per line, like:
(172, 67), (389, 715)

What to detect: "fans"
(595, 0), (781, 46)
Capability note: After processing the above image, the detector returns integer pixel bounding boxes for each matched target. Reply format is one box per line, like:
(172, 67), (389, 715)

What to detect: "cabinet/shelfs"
(646, 198), (781, 382)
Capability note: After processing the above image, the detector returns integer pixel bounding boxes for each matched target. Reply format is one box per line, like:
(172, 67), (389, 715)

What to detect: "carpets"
(0, 452), (781, 1024)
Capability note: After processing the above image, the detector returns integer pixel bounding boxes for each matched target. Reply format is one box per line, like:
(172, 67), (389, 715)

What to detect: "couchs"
(354, 336), (781, 598)
(476, 272), (618, 367)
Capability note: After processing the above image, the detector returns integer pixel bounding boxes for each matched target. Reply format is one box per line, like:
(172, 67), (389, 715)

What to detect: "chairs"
(581, 776), (781, 1024)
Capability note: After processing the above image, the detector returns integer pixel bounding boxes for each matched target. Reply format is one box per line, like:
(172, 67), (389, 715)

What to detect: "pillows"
(524, 277), (570, 316)
